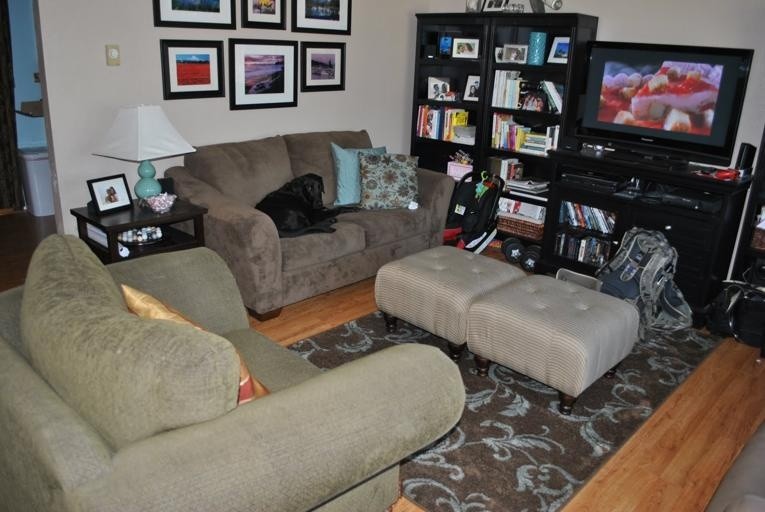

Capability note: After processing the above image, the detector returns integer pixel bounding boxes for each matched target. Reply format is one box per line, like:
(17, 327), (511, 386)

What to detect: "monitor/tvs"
(573, 41), (755, 170)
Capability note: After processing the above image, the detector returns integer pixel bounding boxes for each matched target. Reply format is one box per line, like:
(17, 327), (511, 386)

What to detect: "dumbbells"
(502, 238), (524, 262)
(522, 244), (542, 272)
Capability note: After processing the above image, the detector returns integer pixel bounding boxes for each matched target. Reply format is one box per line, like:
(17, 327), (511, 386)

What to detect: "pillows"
(118, 280), (271, 408)
(192, 135), (294, 206)
(17, 233), (240, 453)
(283, 130), (372, 212)
(358, 151), (420, 209)
(330, 141), (387, 207)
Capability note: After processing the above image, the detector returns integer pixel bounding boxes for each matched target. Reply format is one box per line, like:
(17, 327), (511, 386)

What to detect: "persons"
(457, 42), (474, 55)
(105, 187), (118, 203)
(508, 48), (522, 60)
(432, 83), (447, 100)
(469, 80), (479, 97)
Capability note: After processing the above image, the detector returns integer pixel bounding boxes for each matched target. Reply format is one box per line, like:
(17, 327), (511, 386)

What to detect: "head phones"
(502, 237), (568, 274)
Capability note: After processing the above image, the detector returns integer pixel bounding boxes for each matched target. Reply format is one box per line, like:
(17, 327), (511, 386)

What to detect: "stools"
(373, 245), (527, 360)
(468, 274), (640, 415)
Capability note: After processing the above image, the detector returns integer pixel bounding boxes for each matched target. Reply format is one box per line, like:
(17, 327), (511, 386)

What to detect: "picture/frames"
(87, 173), (134, 217)
(154, 0), (352, 110)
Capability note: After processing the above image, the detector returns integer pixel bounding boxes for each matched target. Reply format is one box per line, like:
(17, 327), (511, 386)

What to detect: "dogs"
(255, 173), (362, 237)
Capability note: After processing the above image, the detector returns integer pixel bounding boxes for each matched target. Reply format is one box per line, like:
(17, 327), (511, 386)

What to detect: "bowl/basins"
(143, 194), (177, 213)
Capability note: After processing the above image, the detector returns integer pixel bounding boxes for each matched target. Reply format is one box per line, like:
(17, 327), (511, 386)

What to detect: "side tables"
(70, 198), (208, 263)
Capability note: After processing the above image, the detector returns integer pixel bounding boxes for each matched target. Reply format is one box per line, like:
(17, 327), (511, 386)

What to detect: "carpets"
(286, 310), (724, 511)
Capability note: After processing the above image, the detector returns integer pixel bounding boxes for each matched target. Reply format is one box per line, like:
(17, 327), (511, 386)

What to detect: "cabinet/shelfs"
(409, 13), (599, 254)
(544, 133), (755, 326)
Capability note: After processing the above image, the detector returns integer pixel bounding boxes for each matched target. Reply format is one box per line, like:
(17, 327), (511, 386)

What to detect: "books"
(488, 157), (549, 241)
(555, 199), (616, 269)
(416, 105), (469, 143)
(491, 69), (560, 158)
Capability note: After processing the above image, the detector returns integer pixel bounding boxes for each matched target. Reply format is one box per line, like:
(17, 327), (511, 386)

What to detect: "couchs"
(2, 245), (465, 511)
(165, 130), (455, 321)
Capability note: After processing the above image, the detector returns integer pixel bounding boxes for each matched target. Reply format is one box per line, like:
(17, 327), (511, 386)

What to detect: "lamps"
(91, 103), (196, 202)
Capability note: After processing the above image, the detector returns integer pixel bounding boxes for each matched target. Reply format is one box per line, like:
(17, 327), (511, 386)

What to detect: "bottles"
(123, 227), (162, 240)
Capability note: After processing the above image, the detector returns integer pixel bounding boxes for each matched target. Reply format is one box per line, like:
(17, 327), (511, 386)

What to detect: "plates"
(120, 242), (157, 246)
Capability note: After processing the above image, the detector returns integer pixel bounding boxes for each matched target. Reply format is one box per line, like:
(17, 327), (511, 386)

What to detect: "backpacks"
(594, 225), (693, 332)
